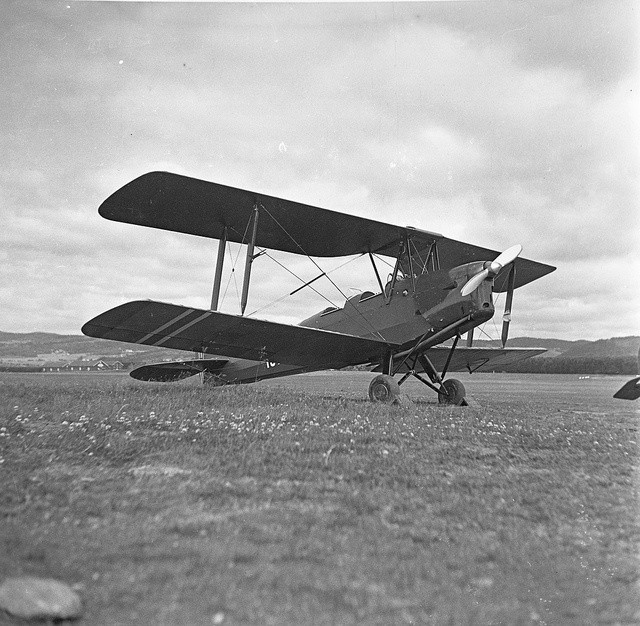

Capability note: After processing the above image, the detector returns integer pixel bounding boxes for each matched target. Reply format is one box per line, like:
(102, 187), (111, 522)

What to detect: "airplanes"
(80, 170), (557, 406)
